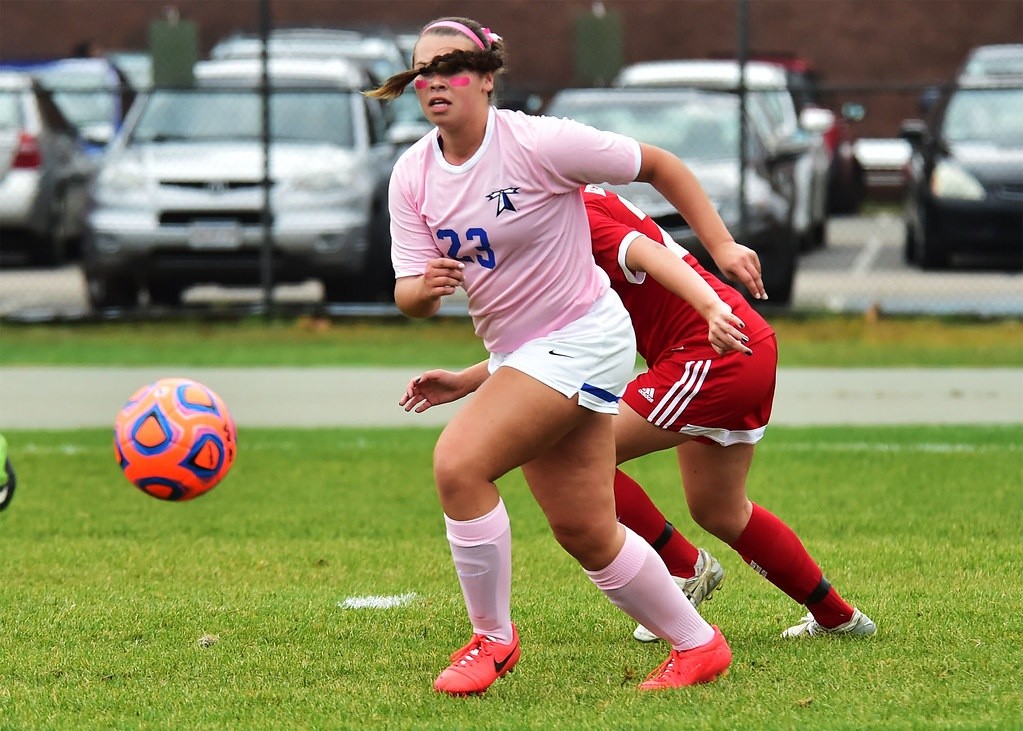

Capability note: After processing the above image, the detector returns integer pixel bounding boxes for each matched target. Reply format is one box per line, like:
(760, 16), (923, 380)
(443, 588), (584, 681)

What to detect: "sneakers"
(433, 621), (521, 698)
(780, 607), (877, 639)
(633, 547), (724, 642)
(637, 624), (732, 691)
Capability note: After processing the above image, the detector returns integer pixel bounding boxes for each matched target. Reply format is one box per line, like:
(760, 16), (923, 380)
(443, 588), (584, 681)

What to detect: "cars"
(536, 53), (863, 311)
(895, 42), (1023, 273)
(0, 25), (502, 312)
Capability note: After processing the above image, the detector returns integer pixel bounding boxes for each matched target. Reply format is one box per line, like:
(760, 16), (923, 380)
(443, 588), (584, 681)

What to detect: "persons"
(398, 183), (884, 641)
(357, 17), (769, 695)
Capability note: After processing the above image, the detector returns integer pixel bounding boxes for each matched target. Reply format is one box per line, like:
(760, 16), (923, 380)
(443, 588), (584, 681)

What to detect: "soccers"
(113, 376), (238, 501)
